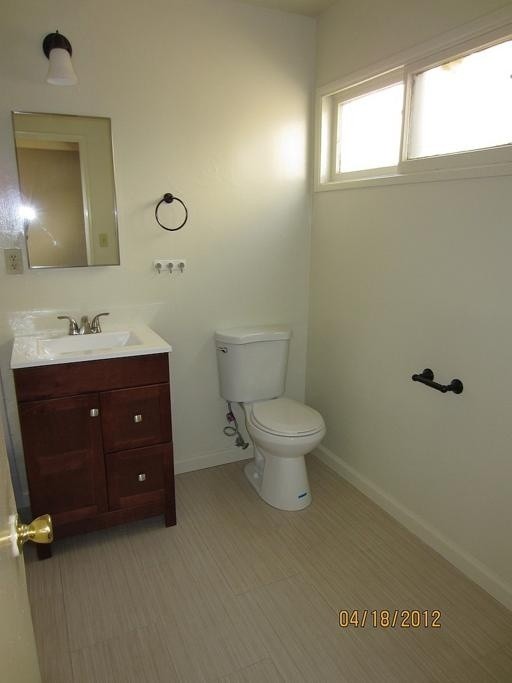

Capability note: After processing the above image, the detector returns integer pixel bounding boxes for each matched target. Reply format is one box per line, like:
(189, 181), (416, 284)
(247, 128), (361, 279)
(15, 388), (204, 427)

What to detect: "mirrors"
(12, 111), (120, 270)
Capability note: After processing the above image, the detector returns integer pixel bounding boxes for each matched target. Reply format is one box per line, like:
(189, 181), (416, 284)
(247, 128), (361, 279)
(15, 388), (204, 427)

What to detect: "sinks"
(36, 330), (144, 361)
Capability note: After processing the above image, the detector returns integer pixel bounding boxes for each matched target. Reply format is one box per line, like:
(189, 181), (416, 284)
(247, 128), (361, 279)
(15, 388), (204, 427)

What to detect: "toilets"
(213, 325), (327, 511)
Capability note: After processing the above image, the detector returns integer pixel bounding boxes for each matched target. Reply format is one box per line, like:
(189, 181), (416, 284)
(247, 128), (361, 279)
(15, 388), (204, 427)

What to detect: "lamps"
(41, 29), (77, 87)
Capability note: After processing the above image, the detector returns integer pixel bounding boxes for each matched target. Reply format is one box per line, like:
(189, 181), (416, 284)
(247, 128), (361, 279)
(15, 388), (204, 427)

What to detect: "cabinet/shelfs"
(11, 352), (177, 558)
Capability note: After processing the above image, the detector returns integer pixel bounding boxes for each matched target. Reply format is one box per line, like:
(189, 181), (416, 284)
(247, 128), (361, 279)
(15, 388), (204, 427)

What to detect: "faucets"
(78, 315), (95, 335)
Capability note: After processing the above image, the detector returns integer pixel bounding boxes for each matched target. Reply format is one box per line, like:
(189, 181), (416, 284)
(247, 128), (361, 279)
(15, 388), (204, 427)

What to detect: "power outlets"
(6, 249), (23, 274)
